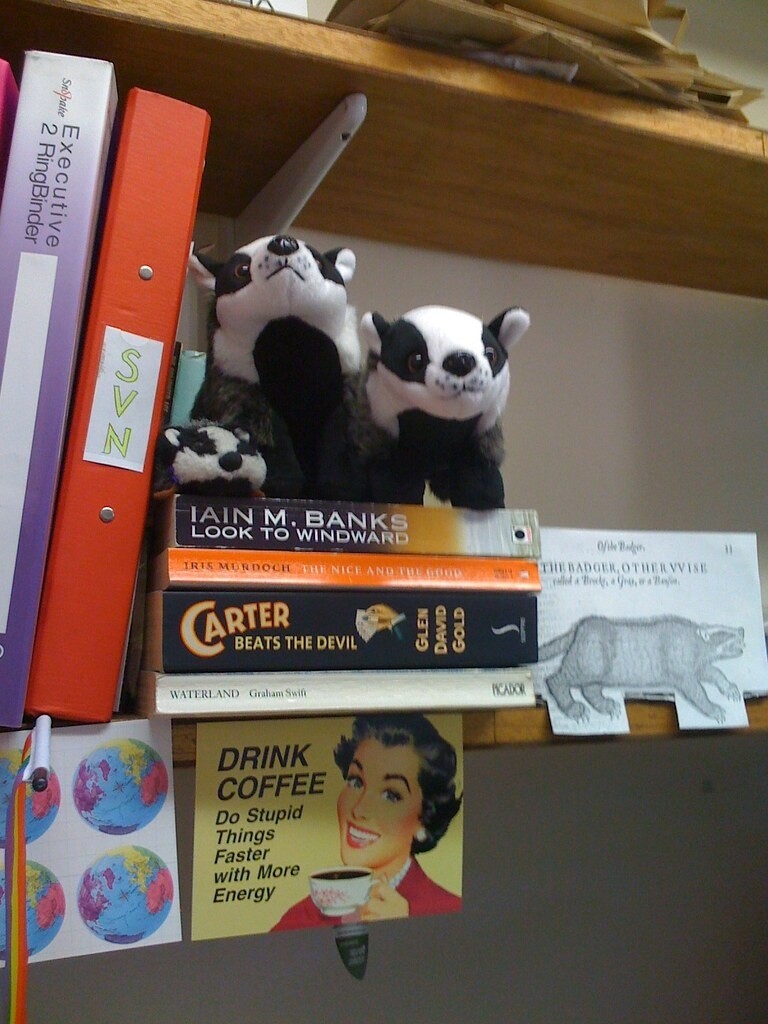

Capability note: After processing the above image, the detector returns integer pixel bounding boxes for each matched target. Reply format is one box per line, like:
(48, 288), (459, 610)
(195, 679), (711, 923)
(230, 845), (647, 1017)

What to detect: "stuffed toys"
(152, 416), (270, 498)
(189, 234), (533, 510)
